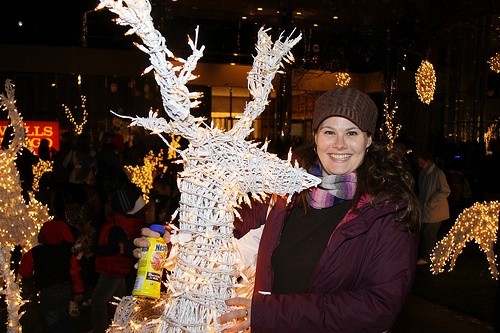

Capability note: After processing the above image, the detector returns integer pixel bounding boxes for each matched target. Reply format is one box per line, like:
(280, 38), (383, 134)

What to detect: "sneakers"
(68, 301), (80, 317)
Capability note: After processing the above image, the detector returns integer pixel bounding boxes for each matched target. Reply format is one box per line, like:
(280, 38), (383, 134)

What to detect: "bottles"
(131, 224), (168, 303)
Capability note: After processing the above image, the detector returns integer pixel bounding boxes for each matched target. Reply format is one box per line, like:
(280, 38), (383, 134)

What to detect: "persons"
(0, 125), (170, 333)
(412, 145), (450, 265)
(133, 87), (421, 333)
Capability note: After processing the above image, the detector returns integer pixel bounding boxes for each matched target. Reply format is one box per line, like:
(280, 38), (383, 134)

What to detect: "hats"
(311, 87), (378, 135)
